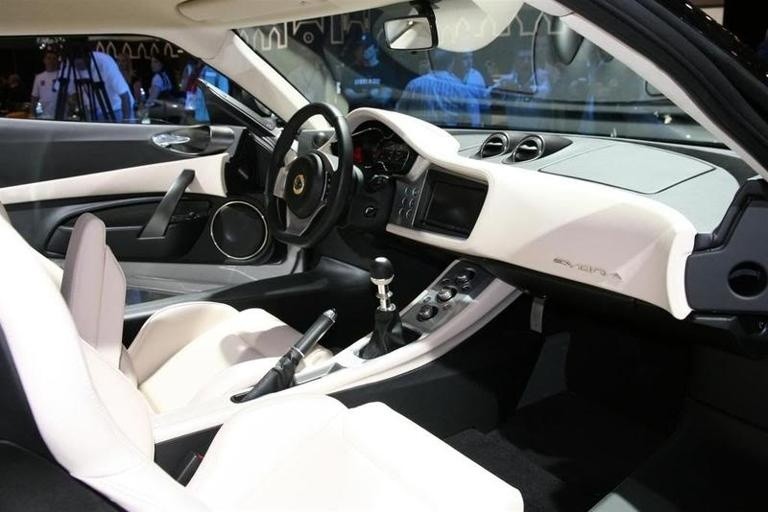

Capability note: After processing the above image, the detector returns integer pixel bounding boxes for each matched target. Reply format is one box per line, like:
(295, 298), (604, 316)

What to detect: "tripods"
(54, 55), (118, 122)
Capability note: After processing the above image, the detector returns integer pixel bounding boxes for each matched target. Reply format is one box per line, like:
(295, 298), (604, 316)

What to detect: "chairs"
(36, 251), (336, 414)
(0, 201), (527, 512)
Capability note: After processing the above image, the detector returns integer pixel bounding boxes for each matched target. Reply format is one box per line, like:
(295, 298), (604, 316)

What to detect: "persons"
(30, 35), (230, 123)
(340, 30), (594, 133)
